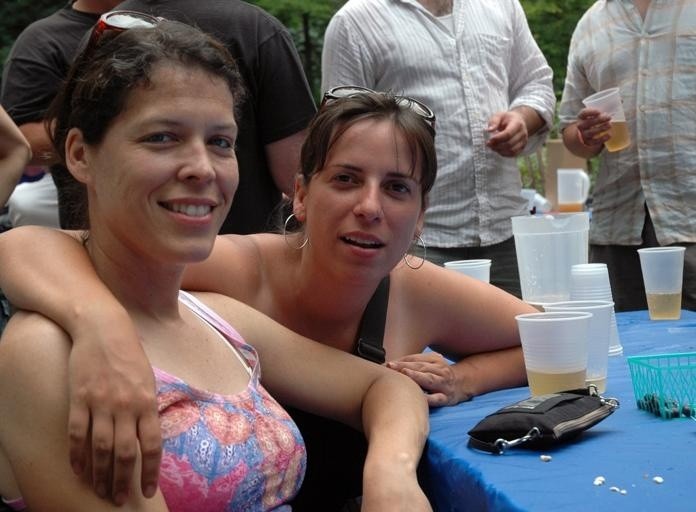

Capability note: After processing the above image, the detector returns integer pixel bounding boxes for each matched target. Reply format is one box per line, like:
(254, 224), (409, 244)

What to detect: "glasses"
(84, 9), (168, 60)
(314, 84), (436, 128)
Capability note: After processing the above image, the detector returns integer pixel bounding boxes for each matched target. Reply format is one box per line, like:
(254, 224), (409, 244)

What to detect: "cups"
(570, 262), (624, 356)
(514, 312), (592, 396)
(444, 259), (492, 284)
(582, 88), (632, 153)
(638, 247), (686, 321)
(544, 301), (615, 396)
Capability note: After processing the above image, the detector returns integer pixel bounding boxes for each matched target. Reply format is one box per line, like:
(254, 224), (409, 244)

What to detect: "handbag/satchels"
(468, 385), (619, 452)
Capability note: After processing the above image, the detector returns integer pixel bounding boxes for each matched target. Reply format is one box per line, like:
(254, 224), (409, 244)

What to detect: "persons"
(1, 16), (436, 512)
(0, 85), (543, 512)
(0, 105), (33, 209)
(5, 165), (60, 228)
(320, 1), (556, 300)
(56, 0), (318, 234)
(559, 0), (696, 313)
(0, 0), (122, 231)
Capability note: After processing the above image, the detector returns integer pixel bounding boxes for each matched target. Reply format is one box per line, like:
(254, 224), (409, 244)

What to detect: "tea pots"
(557, 167), (590, 212)
(511, 212), (590, 303)
